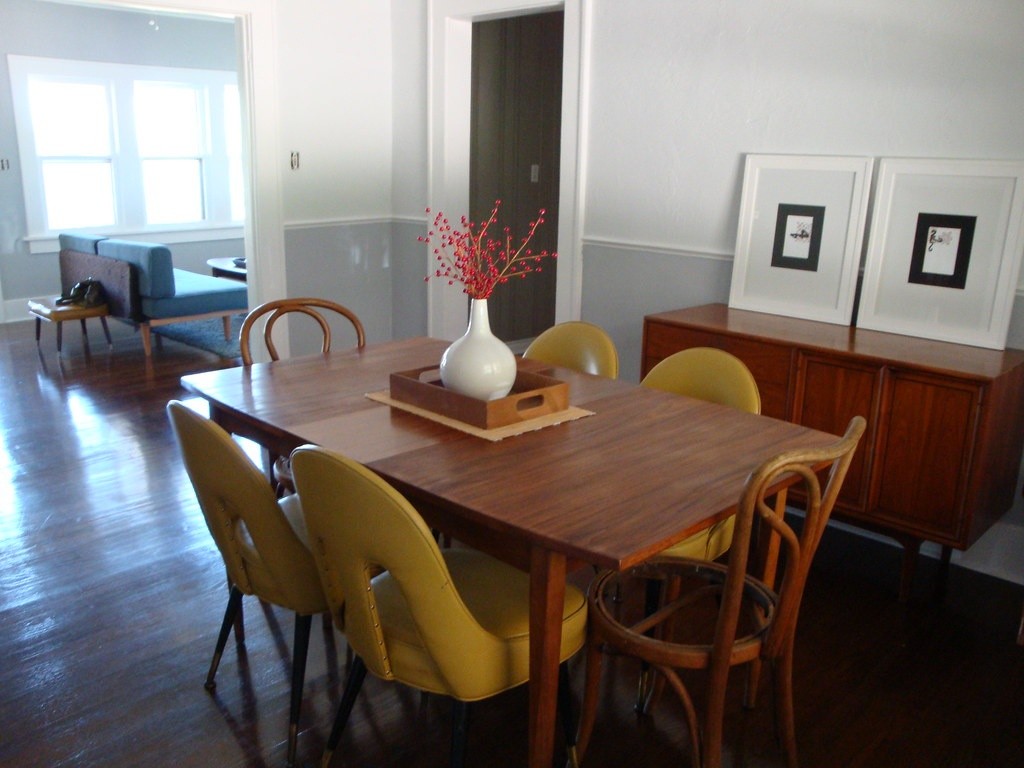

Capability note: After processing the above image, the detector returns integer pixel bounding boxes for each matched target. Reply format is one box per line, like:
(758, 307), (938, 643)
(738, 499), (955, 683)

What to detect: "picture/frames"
(856, 156), (1024, 351)
(728, 154), (874, 326)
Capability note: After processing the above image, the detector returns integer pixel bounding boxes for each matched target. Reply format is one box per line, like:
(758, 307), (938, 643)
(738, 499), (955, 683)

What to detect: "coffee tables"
(206, 256), (247, 283)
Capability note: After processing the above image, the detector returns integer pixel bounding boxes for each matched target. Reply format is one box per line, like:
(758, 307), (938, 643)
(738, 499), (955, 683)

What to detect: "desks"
(180, 335), (844, 768)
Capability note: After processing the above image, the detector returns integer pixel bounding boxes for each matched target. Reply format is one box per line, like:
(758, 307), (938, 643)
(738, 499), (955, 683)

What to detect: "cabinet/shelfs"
(640, 301), (1024, 605)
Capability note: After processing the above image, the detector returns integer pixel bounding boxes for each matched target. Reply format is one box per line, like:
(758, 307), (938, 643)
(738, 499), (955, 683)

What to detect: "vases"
(439, 298), (517, 401)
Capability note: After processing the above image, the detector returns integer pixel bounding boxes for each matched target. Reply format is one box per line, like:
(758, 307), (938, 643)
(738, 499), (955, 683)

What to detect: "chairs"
(167, 401), (433, 768)
(239, 299), (366, 499)
(571, 346), (762, 712)
(289, 445), (590, 768)
(523, 322), (619, 380)
(566, 415), (866, 768)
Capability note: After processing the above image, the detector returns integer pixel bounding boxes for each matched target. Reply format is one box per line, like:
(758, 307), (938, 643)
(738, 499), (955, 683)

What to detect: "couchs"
(60, 232), (249, 356)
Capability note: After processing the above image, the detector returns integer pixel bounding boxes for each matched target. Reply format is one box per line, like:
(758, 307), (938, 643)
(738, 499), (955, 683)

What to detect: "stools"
(27, 295), (114, 357)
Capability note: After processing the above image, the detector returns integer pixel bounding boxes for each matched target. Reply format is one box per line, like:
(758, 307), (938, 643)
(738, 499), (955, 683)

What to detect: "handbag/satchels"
(56, 280), (107, 307)
